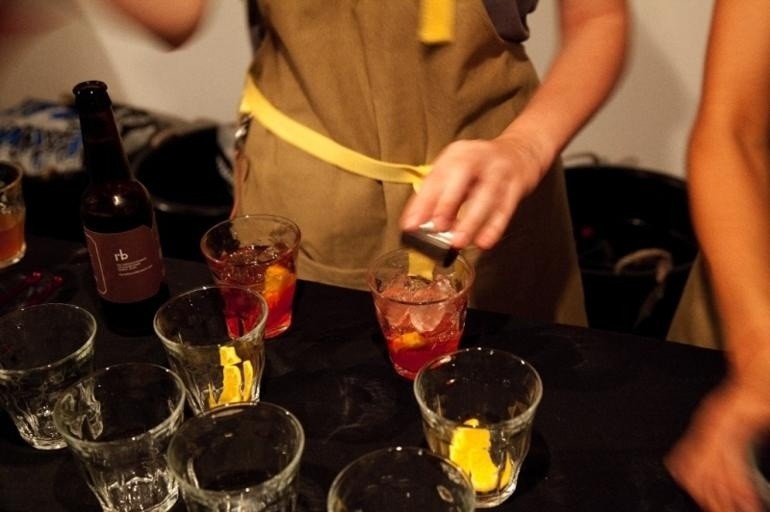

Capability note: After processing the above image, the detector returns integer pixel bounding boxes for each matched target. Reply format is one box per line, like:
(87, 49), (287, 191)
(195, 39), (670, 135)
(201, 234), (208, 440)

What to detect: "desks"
(0, 246), (770, 512)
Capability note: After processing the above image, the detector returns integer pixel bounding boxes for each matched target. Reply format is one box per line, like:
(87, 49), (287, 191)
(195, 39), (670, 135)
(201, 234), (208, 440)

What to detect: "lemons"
(448, 417), (516, 497)
(204, 344), (255, 413)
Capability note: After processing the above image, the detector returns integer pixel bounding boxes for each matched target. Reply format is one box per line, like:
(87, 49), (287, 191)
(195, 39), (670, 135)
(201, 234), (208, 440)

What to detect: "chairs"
(131, 122), (241, 257)
(565, 165), (702, 341)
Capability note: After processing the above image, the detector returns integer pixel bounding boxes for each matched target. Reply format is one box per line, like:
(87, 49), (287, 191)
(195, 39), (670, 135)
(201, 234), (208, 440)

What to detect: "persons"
(662, 0), (770, 512)
(107, 0), (630, 330)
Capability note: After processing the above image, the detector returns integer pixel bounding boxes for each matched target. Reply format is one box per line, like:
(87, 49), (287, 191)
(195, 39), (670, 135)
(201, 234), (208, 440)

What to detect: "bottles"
(73, 80), (172, 338)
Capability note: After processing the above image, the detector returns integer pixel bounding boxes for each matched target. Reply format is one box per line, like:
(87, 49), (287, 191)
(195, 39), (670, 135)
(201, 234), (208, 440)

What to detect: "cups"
(1, 303), (98, 449)
(413, 346), (542, 508)
(0, 160), (27, 267)
(168, 401), (305, 512)
(202, 213), (298, 343)
(153, 285), (269, 417)
(327, 445), (476, 512)
(52, 363), (186, 512)
(366, 248), (477, 381)
